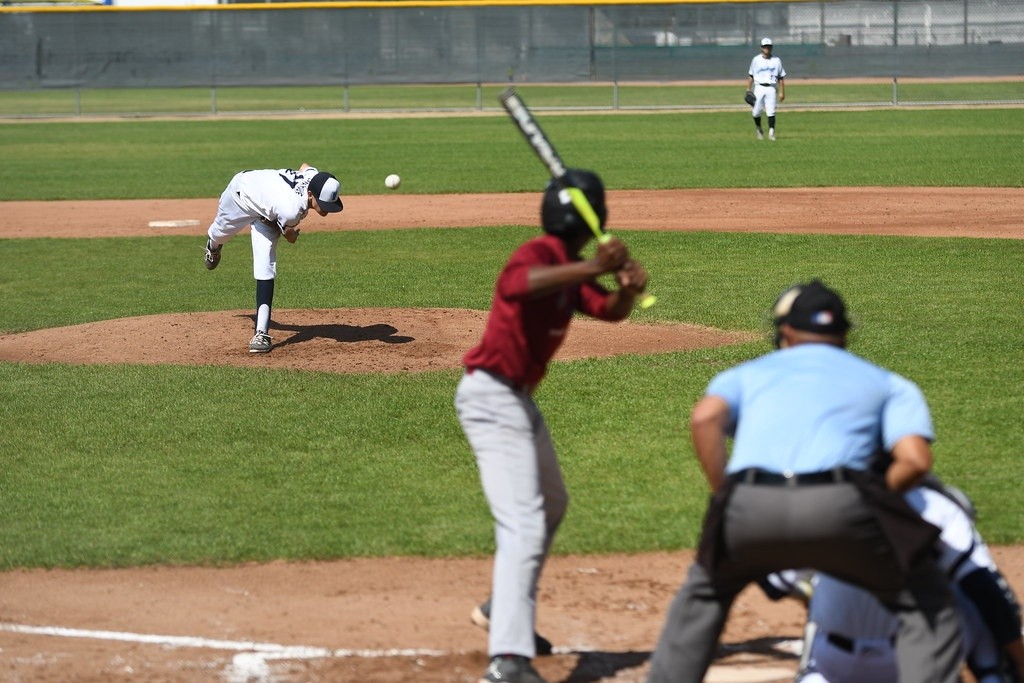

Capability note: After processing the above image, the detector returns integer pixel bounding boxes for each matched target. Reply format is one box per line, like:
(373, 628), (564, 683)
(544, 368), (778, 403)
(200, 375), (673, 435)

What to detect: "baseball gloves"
(744, 91), (756, 107)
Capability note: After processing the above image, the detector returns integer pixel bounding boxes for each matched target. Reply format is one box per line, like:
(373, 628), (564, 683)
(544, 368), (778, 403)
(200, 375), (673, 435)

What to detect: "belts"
(760, 83), (770, 86)
(826, 631), (897, 655)
(237, 168), (252, 197)
(728, 468), (860, 488)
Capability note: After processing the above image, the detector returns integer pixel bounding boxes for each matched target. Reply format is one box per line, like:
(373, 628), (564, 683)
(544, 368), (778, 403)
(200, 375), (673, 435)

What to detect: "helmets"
(539, 169), (605, 240)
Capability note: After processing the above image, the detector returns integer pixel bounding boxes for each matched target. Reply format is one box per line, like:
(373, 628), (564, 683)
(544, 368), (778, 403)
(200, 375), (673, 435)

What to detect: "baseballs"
(384, 174), (400, 190)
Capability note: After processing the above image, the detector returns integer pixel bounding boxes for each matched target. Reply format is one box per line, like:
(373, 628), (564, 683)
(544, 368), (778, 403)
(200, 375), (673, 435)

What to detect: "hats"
(760, 38), (773, 46)
(773, 278), (850, 335)
(308, 171), (344, 213)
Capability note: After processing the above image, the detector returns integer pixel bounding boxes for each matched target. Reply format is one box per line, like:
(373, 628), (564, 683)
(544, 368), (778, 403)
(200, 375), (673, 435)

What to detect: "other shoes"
(768, 134), (776, 140)
(758, 129), (763, 138)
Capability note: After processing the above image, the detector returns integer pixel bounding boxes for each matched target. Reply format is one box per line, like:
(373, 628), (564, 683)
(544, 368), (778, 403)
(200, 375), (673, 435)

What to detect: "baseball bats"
(497, 84), (659, 311)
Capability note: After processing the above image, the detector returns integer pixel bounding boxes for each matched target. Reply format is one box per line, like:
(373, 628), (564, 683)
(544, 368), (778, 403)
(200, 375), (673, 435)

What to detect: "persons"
(645, 279), (970, 682)
(796, 474), (1024, 683)
(451, 170), (650, 683)
(203, 161), (343, 352)
(747, 37), (787, 141)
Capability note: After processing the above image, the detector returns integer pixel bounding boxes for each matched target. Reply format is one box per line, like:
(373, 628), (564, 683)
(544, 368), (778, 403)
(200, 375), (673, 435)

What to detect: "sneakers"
(479, 655), (545, 683)
(204, 236), (223, 270)
(470, 598), (553, 656)
(249, 330), (274, 352)
